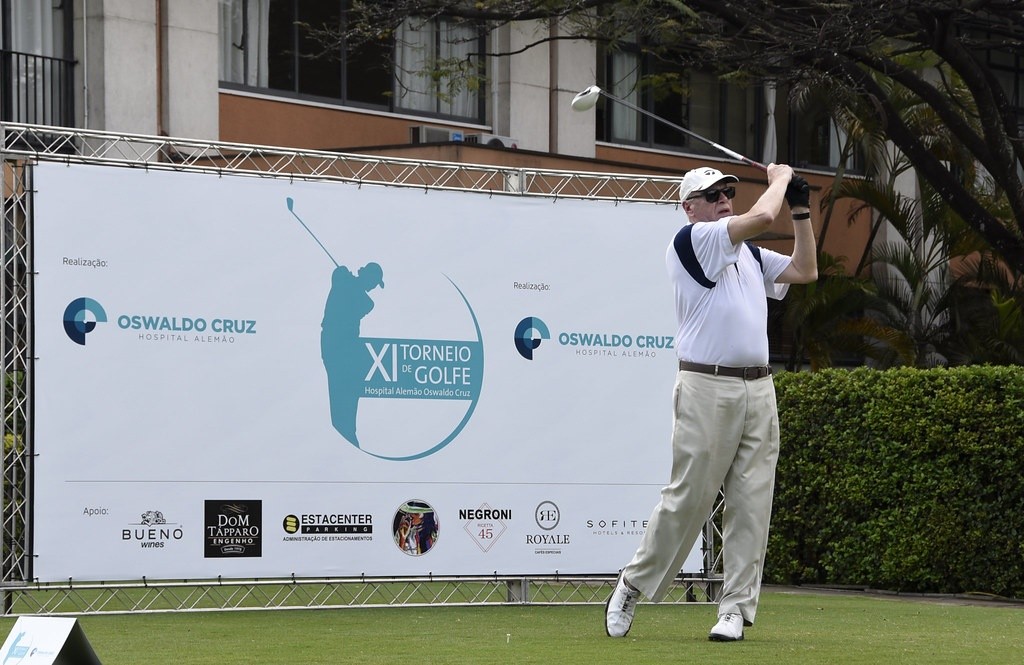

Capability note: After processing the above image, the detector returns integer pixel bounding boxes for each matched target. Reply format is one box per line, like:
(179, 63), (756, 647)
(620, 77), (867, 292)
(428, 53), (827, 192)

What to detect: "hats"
(679, 167), (739, 206)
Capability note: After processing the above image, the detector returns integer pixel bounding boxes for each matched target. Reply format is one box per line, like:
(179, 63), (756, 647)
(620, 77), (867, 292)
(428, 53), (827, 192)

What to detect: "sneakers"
(708, 612), (745, 641)
(605, 566), (641, 638)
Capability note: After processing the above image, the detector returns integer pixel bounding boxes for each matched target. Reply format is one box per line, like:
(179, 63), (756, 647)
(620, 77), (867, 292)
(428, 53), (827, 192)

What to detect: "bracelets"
(792, 212), (810, 220)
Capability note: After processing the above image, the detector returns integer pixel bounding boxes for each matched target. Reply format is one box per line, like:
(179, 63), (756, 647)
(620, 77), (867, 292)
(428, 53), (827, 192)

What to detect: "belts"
(678, 360), (774, 380)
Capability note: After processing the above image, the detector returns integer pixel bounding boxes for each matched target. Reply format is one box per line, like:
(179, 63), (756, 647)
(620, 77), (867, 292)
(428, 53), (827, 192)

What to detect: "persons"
(605, 163), (818, 642)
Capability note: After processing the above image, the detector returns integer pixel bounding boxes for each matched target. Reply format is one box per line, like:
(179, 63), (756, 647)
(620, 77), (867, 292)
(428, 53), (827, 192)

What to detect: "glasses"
(687, 186), (735, 204)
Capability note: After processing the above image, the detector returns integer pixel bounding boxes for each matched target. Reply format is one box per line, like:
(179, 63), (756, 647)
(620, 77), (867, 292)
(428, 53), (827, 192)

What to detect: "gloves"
(784, 174), (810, 220)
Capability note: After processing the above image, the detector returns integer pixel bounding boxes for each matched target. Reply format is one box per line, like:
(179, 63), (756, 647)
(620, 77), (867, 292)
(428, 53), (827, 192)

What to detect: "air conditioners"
(463, 133), (519, 149)
(408, 124), (464, 144)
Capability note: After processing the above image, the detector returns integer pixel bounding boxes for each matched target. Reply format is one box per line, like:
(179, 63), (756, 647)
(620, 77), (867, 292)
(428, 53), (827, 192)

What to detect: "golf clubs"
(569, 84), (823, 192)
